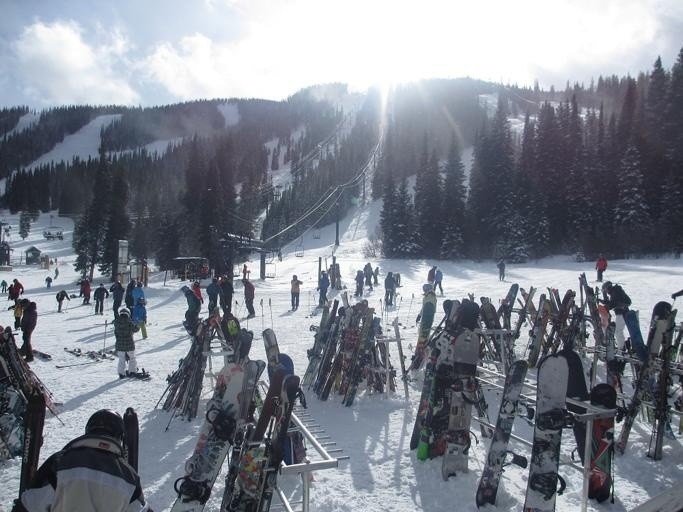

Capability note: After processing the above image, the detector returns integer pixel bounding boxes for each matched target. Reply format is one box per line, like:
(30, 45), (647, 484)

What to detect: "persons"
(7, 299), (23, 330)
(289, 275), (303, 311)
(179, 285), (200, 329)
(205, 276), (220, 315)
(113, 306), (140, 378)
(600, 280), (631, 351)
(129, 297), (148, 338)
(220, 274), (234, 313)
(373, 266), (379, 285)
(364, 265), (373, 291)
(363, 263), (373, 286)
(190, 281), (203, 306)
(384, 271), (396, 305)
(16, 408), (152, 512)
(433, 269), (443, 294)
(316, 272), (329, 307)
(0, 263), (143, 317)
(427, 265), (437, 285)
(17, 298), (37, 362)
(354, 270), (364, 296)
(414, 282), (437, 352)
(240, 278), (255, 319)
(496, 259), (504, 281)
(595, 254), (607, 282)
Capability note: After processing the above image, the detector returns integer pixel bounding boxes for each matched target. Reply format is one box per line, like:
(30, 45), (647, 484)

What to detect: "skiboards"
(446, 283), (682, 460)
(302, 293), (411, 406)
(56, 345), (150, 383)
(156, 318), (208, 430)
(0, 321), (62, 468)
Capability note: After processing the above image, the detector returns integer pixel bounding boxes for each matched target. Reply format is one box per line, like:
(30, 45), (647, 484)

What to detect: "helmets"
(85, 409), (123, 441)
(120, 308), (131, 319)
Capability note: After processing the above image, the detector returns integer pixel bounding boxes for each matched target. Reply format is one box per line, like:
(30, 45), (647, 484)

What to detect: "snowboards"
(409, 328), (459, 461)
(410, 290), (437, 369)
(556, 347), (600, 468)
(474, 361), (528, 511)
(441, 330), (479, 479)
(522, 354), (569, 512)
(170, 325), (303, 512)
(587, 383), (619, 505)
